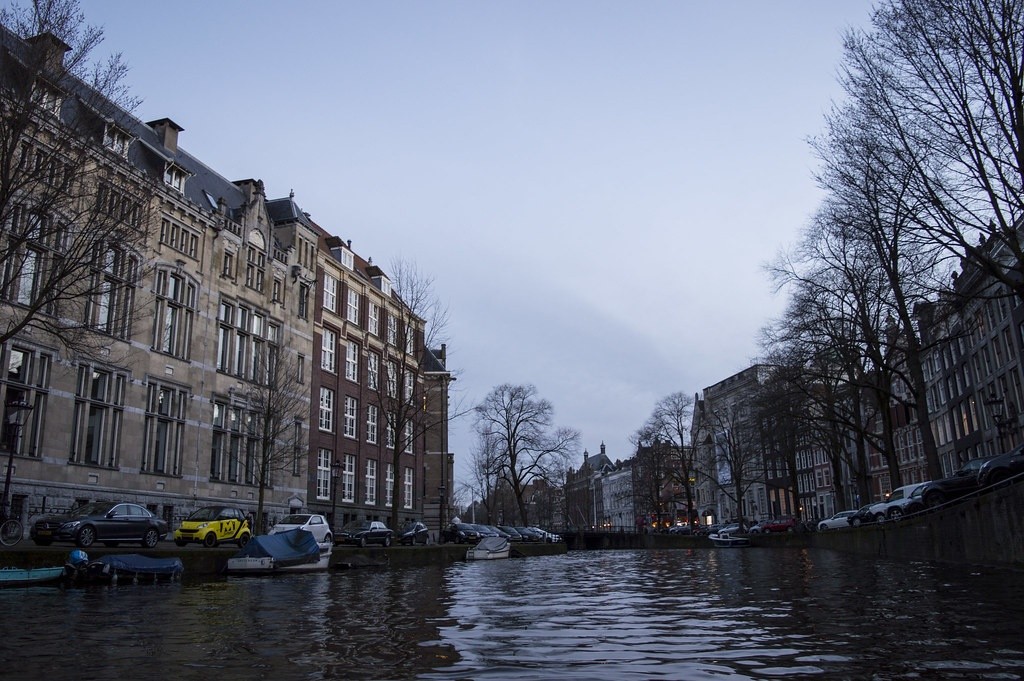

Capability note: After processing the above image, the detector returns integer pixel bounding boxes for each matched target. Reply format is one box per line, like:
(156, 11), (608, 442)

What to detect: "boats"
(66, 550), (184, 585)
(708, 534), (752, 548)
(466, 537), (511, 560)
(0, 566), (67, 586)
(227, 526), (332, 573)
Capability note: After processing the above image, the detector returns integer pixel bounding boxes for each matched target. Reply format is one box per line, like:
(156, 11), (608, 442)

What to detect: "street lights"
(593, 474), (602, 533)
(0, 395), (35, 535)
(983, 392), (1005, 454)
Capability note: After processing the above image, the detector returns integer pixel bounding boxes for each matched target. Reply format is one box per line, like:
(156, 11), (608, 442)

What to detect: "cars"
(332, 520), (396, 548)
(28, 501), (168, 548)
(174, 505), (252, 549)
(439, 523), (565, 544)
(818, 441), (1024, 531)
(268, 514), (333, 543)
(391, 521), (430, 546)
(658, 516), (799, 536)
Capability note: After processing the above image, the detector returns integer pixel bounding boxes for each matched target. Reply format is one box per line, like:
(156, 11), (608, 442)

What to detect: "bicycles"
(0, 501), (24, 547)
